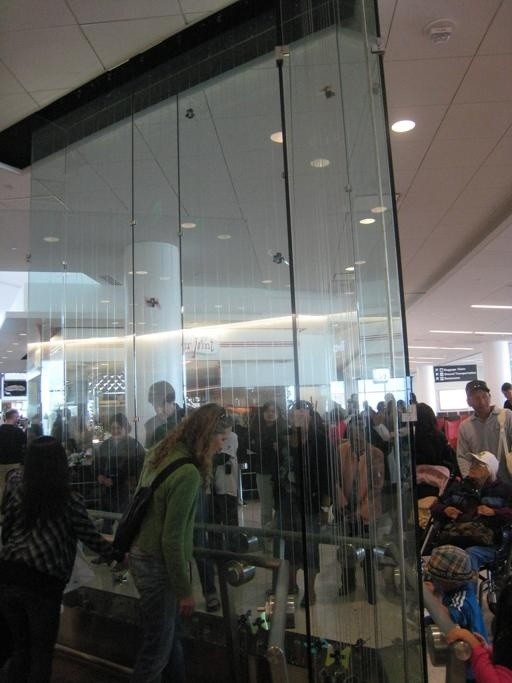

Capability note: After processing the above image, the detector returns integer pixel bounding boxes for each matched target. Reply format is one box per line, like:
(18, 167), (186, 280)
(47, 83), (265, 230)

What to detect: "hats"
(465, 380), (491, 395)
(465, 451), (500, 475)
(423, 545), (477, 585)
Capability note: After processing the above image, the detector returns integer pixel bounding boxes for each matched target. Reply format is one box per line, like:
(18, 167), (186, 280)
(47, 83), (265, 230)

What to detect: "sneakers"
(300, 591), (318, 608)
(338, 584), (355, 595)
(365, 586), (377, 605)
(206, 593), (220, 611)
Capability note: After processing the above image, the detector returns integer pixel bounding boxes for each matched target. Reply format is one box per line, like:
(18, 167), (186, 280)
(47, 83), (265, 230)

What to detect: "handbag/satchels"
(112, 486), (154, 554)
(437, 510), (496, 549)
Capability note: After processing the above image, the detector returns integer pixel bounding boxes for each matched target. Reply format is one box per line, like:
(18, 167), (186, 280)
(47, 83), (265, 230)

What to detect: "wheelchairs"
(382, 478), (510, 626)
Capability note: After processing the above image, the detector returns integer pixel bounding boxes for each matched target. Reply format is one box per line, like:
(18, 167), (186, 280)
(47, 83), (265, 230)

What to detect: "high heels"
(91, 557), (114, 566)
